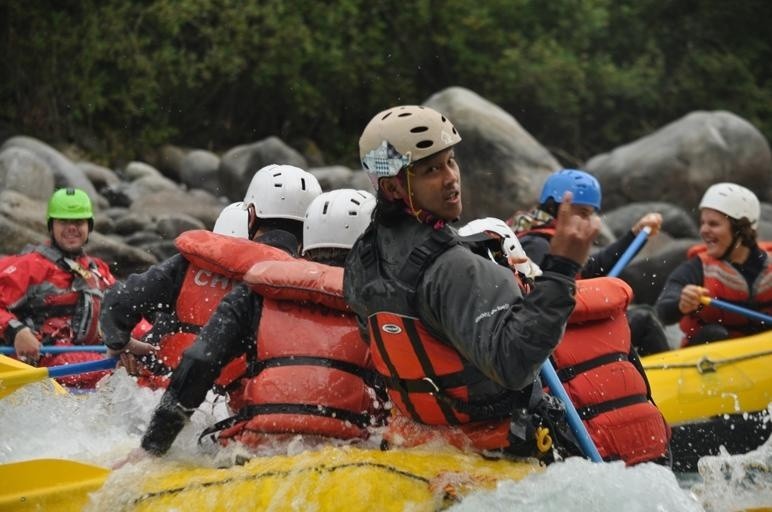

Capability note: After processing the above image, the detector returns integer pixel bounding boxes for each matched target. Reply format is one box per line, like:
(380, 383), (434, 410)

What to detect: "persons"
(100, 162), (322, 391)
(0, 186), (152, 390)
(112, 188), (388, 471)
(504, 167), (671, 357)
(457, 216), (673, 470)
(654, 180), (772, 349)
(343, 105), (601, 467)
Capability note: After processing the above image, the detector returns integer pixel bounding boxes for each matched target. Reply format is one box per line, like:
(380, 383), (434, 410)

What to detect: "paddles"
(0, 353), (117, 398)
(0, 459), (113, 511)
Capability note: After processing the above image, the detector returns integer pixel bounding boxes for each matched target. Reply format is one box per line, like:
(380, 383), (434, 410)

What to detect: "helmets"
(458, 216), (544, 279)
(242, 164), (323, 223)
(699, 181), (761, 231)
(538, 168), (602, 211)
(301, 188), (379, 256)
(212, 201), (249, 239)
(47, 188), (95, 233)
(359, 104), (463, 195)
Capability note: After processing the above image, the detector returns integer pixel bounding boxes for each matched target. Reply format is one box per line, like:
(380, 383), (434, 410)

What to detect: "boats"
(641, 328), (772, 472)
(113, 448), (542, 512)
